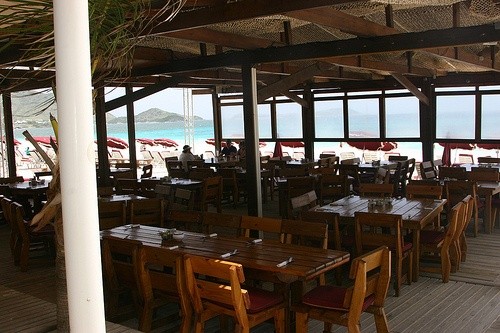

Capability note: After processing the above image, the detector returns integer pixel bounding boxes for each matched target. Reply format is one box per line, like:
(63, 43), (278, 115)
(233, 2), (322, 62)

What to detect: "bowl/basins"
(173, 233), (184, 240)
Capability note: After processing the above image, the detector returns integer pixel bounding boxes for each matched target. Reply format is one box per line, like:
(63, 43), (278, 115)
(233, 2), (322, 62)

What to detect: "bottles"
(368, 199), (383, 208)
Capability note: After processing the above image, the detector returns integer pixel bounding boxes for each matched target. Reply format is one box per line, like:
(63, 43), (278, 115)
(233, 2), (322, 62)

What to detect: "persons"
(218, 140), (236, 159)
(24, 147), (30, 155)
(177, 145), (198, 173)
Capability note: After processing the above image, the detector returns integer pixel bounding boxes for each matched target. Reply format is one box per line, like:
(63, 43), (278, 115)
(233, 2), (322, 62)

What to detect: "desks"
(286, 161), (318, 166)
(308, 194), (447, 283)
(9, 181), (50, 211)
(477, 180), (500, 234)
(359, 162), (397, 169)
(98, 194), (148, 206)
(162, 179), (202, 186)
(100, 224), (351, 333)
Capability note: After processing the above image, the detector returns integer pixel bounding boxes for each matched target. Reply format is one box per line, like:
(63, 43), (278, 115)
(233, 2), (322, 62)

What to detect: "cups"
(161, 228), (176, 240)
(384, 197), (391, 204)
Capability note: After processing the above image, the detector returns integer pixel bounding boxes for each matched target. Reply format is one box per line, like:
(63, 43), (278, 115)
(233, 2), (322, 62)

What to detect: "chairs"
(0, 150), (500, 333)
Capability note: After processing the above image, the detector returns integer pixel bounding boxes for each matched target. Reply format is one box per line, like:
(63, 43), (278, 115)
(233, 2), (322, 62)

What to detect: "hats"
(182, 145), (192, 152)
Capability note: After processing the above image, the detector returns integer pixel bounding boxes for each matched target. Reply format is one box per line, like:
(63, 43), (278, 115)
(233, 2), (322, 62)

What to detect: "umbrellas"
(477, 143), (500, 159)
(278, 140), (305, 159)
(204, 137), (266, 153)
(0, 134), (178, 168)
(438, 142), (476, 166)
(347, 140), (397, 164)
